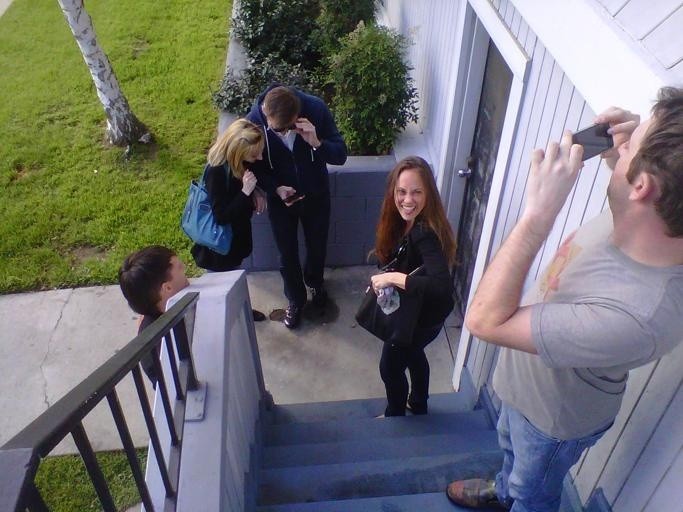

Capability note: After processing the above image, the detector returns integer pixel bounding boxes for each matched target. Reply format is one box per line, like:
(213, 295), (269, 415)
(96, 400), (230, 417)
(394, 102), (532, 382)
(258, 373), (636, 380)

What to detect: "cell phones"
(572, 122), (613, 162)
(281, 192), (308, 208)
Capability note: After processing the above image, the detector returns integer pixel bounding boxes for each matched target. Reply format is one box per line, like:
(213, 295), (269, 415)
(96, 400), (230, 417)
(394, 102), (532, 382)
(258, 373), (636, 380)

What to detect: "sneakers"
(284, 298), (304, 329)
(308, 285), (328, 308)
(446, 478), (506, 509)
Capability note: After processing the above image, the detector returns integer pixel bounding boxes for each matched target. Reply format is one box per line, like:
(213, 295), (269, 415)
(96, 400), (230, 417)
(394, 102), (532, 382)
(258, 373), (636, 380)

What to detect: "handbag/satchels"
(180, 179), (232, 255)
(356, 271), (423, 349)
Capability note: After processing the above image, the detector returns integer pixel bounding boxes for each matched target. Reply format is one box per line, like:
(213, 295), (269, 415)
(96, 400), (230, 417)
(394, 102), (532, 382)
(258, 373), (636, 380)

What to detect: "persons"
(116, 245), (191, 399)
(348, 152), (457, 420)
(243, 85), (347, 331)
(190, 118), (268, 324)
(445, 87), (681, 511)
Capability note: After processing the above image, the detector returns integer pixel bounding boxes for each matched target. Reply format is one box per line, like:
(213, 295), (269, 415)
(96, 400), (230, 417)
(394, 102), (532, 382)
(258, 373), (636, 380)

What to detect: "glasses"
(270, 122), (299, 132)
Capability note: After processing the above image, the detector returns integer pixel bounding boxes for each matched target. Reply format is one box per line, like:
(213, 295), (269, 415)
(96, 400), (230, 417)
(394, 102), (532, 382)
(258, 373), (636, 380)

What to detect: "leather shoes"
(253, 309), (265, 320)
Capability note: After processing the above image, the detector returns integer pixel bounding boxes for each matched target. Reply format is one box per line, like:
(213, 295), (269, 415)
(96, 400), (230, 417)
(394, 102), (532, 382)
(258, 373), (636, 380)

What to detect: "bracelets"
(311, 142), (321, 151)
